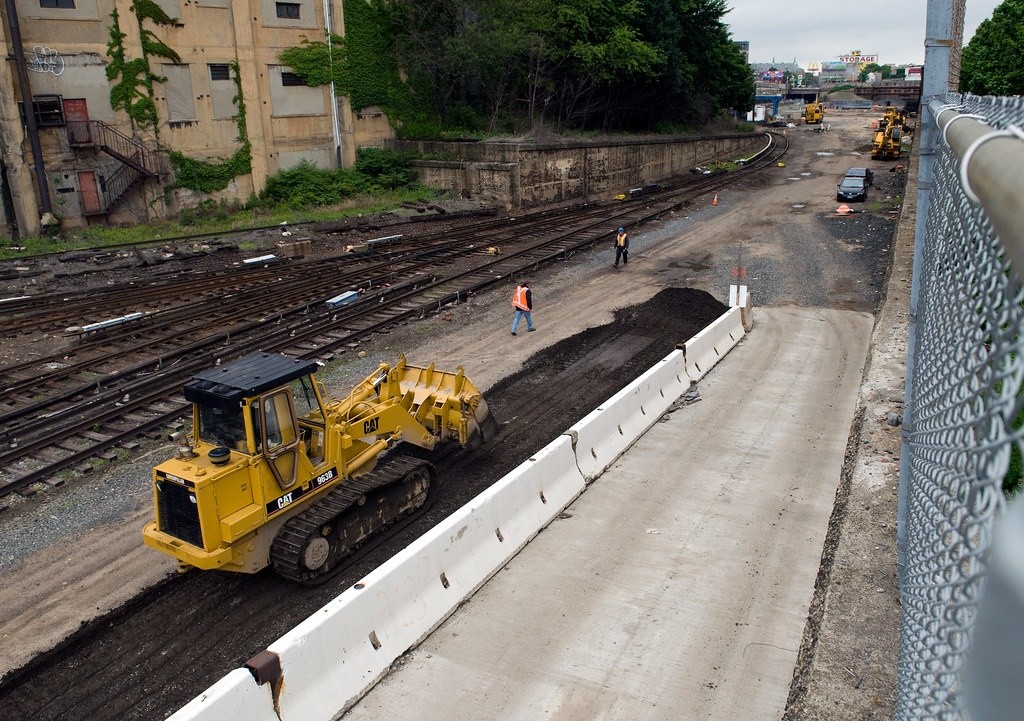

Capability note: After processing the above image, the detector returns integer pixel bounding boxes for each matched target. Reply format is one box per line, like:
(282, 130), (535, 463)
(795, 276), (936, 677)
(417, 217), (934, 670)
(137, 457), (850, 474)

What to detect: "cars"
(835, 176), (870, 203)
(845, 167), (875, 184)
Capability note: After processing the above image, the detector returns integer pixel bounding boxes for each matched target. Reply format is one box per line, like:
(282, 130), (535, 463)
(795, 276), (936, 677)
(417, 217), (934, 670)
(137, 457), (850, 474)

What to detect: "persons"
(511, 281), (536, 336)
(612, 227), (629, 268)
(820, 121), (826, 133)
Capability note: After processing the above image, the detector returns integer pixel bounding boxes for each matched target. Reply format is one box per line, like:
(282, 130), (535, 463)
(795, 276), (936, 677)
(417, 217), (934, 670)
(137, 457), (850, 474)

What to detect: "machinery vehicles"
(805, 93), (824, 123)
(141, 349), (509, 588)
(879, 107), (908, 130)
(870, 123), (902, 161)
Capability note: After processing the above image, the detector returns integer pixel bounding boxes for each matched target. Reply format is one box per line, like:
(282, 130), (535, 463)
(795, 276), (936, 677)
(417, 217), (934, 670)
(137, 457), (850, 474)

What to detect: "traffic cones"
(711, 192), (719, 206)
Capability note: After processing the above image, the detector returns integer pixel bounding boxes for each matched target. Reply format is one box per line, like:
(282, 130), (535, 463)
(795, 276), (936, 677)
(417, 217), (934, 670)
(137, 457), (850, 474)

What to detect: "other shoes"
(527, 328), (536, 332)
(612, 264), (618, 269)
(623, 263), (627, 265)
(511, 332), (517, 336)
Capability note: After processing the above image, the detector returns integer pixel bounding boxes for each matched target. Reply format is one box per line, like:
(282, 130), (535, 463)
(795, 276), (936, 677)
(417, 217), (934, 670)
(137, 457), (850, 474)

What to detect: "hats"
(618, 227), (624, 232)
